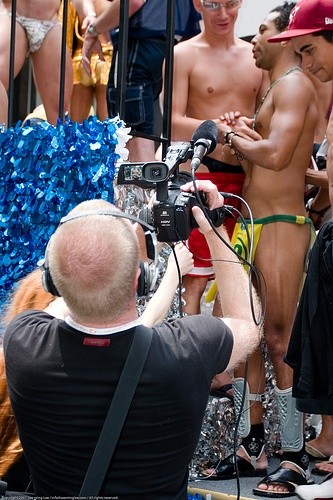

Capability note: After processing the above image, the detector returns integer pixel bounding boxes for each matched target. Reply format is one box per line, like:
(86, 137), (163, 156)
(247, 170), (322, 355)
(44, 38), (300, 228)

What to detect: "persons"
(197, 3), (319, 498)
(3, 180), (263, 500)
(0, 0), (96, 130)
(0, 243), (194, 477)
(82, 0), (202, 162)
(161, 0), (271, 316)
(268, 0), (333, 476)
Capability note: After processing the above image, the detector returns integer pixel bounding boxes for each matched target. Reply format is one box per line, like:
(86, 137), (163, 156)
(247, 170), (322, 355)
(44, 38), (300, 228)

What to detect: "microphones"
(189, 119), (218, 170)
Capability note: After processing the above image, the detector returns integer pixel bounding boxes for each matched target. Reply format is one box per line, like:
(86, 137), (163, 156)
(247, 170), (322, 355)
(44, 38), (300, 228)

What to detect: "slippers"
(304, 443), (329, 461)
(311, 462), (333, 477)
(199, 454), (267, 480)
(252, 466), (314, 497)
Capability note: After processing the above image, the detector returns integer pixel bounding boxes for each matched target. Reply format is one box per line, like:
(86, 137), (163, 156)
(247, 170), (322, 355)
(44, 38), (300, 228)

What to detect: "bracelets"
(223, 131), (245, 161)
(89, 22), (100, 36)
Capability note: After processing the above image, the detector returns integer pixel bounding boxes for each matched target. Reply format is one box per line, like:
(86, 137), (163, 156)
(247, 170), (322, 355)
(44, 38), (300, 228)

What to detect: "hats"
(266, 0), (333, 43)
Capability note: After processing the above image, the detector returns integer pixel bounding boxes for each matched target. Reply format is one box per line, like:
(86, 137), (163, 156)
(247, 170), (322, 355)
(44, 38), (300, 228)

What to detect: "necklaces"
(252, 67), (301, 131)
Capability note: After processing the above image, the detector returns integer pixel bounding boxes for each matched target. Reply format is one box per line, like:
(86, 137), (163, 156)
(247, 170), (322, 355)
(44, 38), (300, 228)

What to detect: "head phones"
(37, 210), (158, 298)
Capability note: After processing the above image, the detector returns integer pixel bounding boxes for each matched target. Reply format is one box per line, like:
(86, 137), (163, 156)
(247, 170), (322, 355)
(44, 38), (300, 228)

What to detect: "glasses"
(199, 0), (241, 11)
